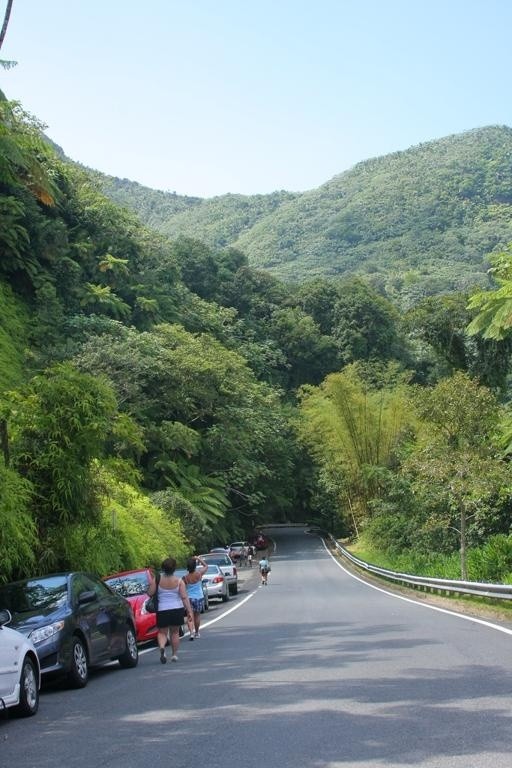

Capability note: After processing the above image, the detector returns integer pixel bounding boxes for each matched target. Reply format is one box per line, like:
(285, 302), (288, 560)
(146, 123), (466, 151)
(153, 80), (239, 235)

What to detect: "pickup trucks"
(195, 552), (238, 596)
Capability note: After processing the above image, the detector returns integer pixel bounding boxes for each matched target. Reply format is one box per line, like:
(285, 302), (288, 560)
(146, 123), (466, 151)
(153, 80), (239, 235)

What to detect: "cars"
(101, 568), (185, 646)
(195, 565), (230, 602)
(0, 608), (42, 717)
(156, 570), (209, 614)
(209, 542), (256, 562)
(0, 570), (138, 689)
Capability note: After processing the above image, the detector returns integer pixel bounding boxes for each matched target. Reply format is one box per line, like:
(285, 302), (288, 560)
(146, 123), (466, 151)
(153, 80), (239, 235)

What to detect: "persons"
(147, 559), (194, 664)
(182, 555), (209, 640)
(224, 544), (254, 567)
(259, 557), (268, 586)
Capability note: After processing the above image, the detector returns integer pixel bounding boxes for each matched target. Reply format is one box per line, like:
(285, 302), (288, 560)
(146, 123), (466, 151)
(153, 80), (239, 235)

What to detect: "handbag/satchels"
(144, 598), (159, 612)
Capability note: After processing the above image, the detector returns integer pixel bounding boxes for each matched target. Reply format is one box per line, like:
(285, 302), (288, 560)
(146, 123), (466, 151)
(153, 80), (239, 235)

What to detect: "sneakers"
(160, 633), (200, 663)
